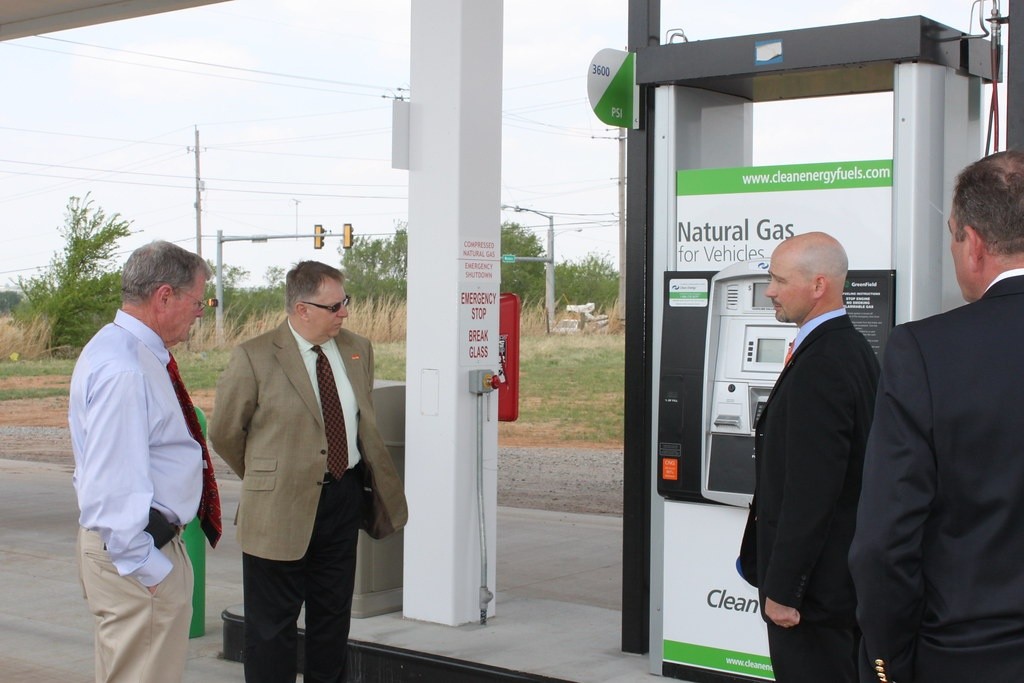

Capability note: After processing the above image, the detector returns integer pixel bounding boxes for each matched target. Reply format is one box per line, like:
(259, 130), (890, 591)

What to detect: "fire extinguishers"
(498, 292), (522, 422)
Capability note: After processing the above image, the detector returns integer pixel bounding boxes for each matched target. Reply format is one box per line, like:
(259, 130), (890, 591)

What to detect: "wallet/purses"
(143, 505), (177, 550)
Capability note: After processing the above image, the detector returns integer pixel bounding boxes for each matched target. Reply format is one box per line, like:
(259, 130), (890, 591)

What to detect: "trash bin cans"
(349, 378), (409, 619)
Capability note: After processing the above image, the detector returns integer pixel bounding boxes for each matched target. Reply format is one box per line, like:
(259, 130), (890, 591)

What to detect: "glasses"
(151, 280), (207, 311)
(299, 295), (351, 313)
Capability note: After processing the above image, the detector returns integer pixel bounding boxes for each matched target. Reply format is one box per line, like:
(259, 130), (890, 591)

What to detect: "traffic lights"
(314, 225), (324, 248)
(209, 298), (218, 306)
(344, 223), (355, 248)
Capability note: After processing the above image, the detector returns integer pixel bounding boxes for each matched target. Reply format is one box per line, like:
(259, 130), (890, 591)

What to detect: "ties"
(164, 345), (222, 551)
(785, 339), (796, 367)
(309, 345), (350, 481)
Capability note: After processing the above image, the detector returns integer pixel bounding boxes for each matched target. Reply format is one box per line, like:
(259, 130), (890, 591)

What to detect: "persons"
(850, 146), (1023, 683)
(67, 238), (213, 683)
(208, 260), (411, 683)
(738, 230), (881, 683)
